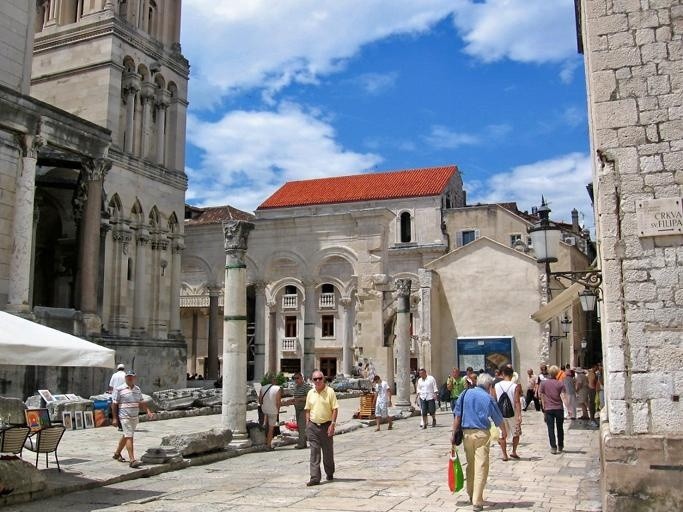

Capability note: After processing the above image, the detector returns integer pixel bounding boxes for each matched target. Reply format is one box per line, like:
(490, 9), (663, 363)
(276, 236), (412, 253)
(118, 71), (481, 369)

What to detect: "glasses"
(312, 377), (324, 383)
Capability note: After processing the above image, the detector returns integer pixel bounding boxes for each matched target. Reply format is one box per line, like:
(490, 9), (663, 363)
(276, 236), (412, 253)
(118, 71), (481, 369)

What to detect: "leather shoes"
(294, 442), (307, 449)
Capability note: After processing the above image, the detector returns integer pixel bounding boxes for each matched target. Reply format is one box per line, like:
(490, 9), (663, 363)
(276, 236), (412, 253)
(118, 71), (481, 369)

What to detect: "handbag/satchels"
(453, 429), (465, 446)
(448, 448), (464, 493)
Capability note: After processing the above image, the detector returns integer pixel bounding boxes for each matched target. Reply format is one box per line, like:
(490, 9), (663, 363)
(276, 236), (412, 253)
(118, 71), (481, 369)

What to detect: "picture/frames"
(62, 410), (73, 431)
(38, 389), (57, 404)
(54, 394), (68, 402)
(82, 410), (94, 428)
(74, 411), (84, 430)
(65, 393), (78, 401)
(24, 408), (53, 432)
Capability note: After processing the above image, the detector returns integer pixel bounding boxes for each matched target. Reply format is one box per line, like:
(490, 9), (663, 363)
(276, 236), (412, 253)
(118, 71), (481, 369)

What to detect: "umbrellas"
(0, 310), (115, 370)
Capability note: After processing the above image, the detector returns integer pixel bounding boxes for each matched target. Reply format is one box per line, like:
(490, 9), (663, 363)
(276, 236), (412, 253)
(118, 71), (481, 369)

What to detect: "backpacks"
(496, 382), (514, 418)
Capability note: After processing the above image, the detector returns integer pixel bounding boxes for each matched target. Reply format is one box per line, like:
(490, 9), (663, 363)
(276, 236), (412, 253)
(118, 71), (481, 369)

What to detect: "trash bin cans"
(246, 423), (267, 445)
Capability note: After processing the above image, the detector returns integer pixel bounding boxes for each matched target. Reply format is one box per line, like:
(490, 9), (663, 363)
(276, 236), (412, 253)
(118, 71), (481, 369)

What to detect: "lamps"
(529, 201), (604, 284)
(576, 288), (602, 320)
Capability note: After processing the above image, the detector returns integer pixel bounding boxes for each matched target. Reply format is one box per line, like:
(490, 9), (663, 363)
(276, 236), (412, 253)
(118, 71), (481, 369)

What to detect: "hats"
(116, 364), (124, 369)
(125, 370), (136, 377)
(574, 366), (583, 373)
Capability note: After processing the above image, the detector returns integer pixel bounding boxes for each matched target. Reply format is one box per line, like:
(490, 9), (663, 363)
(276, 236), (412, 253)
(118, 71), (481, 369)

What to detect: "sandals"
(503, 455), (509, 461)
(112, 452), (126, 462)
(129, 460), (143, 468)
(510, 454), (521, 459)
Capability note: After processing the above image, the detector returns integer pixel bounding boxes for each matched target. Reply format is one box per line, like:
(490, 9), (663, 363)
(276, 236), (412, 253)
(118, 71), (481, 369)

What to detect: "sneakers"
(306, 476), (320, 486)
(473, 505), (484, 512)
(326, 474), (334, 480)
(589, 420), (599, 427)
(432, 419), (436, 428)
(550, 447), (563, 455)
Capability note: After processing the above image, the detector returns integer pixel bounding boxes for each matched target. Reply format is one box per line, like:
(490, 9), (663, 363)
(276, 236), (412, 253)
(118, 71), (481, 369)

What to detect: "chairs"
(0, 424), (31, 459)
(22, 425), (66, 469)
